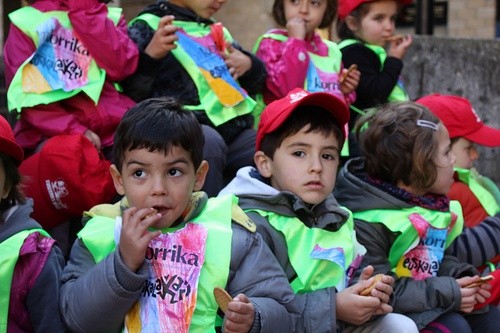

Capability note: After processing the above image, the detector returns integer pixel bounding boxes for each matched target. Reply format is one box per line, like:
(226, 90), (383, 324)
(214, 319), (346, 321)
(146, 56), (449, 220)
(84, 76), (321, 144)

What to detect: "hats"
(18, 135), (116, 225)
(414, 92), (497, 146)
(255, 86), (350, 153)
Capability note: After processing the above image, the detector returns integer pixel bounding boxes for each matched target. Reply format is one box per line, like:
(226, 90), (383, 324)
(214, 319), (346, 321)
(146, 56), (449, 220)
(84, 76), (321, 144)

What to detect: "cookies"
(214, 287), (237, 312)
(359, 275), (382, 295)
(144, 210), (158, 218)
(466, 276), (494, 287)
(385, 34), (402, 40)
(340, 64), (357, 83)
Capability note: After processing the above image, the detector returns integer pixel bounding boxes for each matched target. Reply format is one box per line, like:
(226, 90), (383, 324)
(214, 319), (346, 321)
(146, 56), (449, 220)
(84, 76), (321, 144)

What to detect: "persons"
(252, 0), (361, 170)
(0, 0), (268, 258)
(334, 92), (500, 333)
(59, 97), (301, 333)
(335, 0), (412, 158)
(217, 88), (419, 333)
(0, 115), (67, 333)
